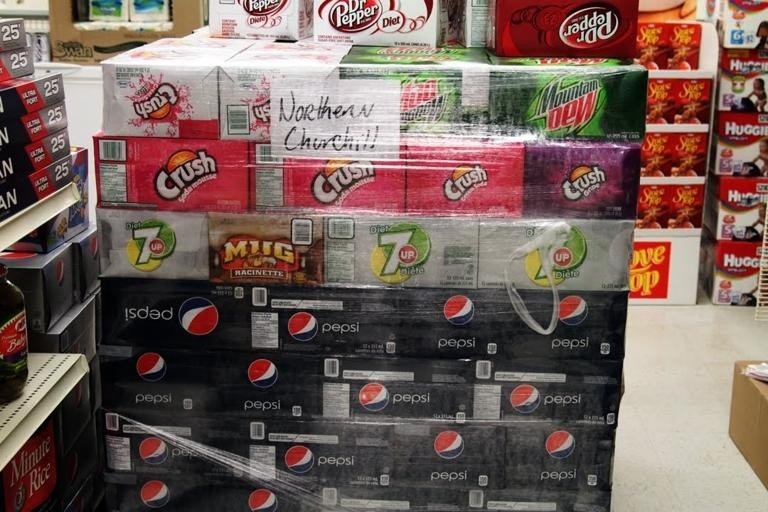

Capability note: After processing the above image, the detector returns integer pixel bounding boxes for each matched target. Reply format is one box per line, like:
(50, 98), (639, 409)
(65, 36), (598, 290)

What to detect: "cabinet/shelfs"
(0, 180), (90, 471)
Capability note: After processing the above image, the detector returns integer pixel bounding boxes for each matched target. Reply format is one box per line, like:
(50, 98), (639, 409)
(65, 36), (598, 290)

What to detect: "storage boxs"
(48, 0), (204, 66)
(728, 359), (767, 492)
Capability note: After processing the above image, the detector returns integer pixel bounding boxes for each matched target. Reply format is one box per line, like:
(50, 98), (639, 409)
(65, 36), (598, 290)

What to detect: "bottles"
(0, 264), (29, 402)
